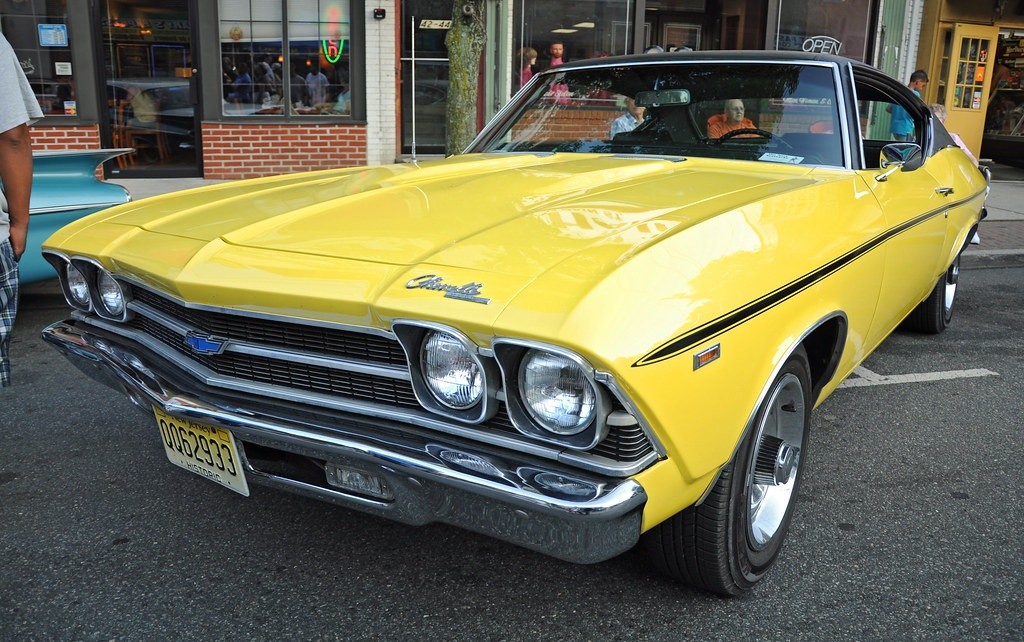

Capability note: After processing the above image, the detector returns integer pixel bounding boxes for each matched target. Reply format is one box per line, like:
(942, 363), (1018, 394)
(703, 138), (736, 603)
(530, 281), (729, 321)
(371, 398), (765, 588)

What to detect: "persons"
(886, 70), (928, 142)
(0, 34), (45, 389)
(707, 99), (760, 138)
(118, 76), (158, 128)
(223, 56), (351, 113)
(610, 97), (646, 140)
(515, 47), (537, 87)
(547, 40), (571, 105)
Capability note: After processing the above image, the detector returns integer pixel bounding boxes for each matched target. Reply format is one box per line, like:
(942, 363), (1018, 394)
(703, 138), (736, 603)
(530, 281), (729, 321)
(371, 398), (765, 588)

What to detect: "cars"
(40, 50), (988, 595)
(105, 79), (270, 163)
(404, 79), (450, 134)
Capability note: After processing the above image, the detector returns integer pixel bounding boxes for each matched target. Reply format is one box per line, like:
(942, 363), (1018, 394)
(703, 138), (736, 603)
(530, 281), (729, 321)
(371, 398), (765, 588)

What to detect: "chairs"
(41, 98), (174, 168)
(778, 130), (841, 161)
(611, 130), (677, 147)
